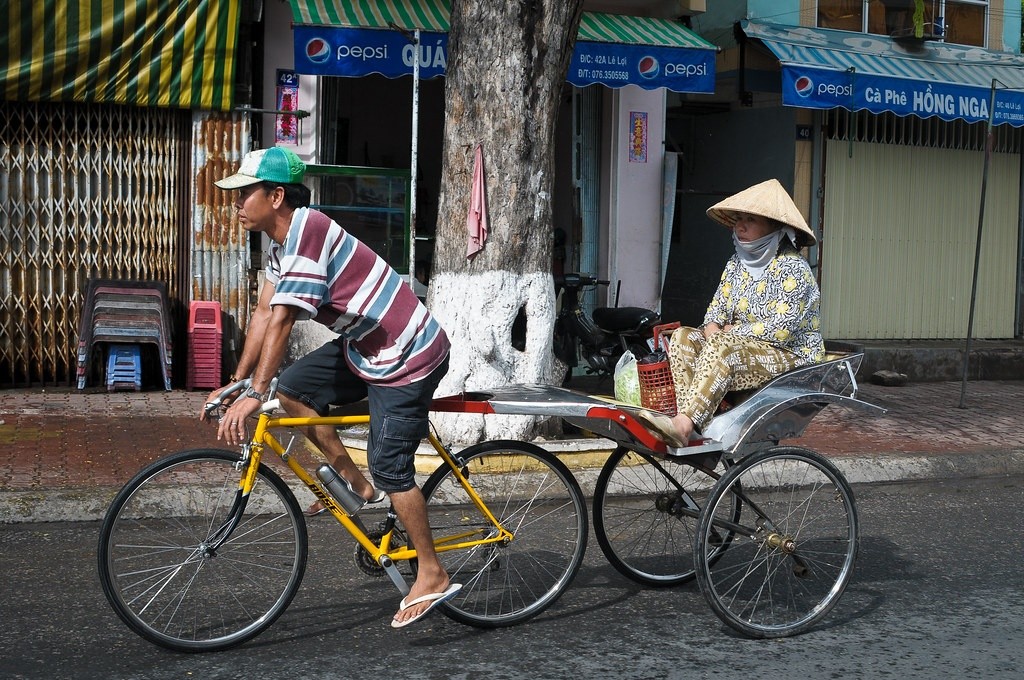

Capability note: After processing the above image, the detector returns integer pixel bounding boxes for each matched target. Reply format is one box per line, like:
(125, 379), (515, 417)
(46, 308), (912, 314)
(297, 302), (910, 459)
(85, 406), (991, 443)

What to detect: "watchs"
(246, 387), (263, 402)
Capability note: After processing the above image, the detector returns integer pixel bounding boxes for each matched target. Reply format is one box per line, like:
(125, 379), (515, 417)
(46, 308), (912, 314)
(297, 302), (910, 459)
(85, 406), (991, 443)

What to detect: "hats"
(706, 179), (817, 247)
(214, 146), (307, 190)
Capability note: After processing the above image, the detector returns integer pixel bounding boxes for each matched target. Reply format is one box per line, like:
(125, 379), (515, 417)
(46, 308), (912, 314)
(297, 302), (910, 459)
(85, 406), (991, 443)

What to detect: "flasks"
(315, 463), (364, 516)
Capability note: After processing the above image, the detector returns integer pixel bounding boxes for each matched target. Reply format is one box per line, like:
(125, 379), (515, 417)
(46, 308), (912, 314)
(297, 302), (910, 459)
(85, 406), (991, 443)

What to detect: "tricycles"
(96, 340), (889, 655)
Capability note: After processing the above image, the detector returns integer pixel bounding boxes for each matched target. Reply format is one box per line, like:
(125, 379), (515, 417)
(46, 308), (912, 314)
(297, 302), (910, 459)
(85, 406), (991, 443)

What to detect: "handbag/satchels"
(636, 321), (681, 415)
(614, 350), (643, 407)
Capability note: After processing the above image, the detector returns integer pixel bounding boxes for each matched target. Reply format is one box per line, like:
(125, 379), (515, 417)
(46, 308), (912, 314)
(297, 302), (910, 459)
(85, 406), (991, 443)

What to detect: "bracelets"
(230, 375), (239, 383)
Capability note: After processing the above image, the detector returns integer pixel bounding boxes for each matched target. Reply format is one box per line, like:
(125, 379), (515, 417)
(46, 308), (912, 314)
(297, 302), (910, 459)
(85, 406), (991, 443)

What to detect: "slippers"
(309, 489), (385, 516)
(395, 582), (463, 628)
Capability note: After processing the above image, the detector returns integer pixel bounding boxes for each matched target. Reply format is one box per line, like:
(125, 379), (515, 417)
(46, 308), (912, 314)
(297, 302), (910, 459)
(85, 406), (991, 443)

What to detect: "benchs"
(562, 348), (864, 456)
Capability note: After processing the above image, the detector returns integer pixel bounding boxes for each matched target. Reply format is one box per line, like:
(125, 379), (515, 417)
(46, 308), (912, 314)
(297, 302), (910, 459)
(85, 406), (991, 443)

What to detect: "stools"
(76, 278), (224, 392)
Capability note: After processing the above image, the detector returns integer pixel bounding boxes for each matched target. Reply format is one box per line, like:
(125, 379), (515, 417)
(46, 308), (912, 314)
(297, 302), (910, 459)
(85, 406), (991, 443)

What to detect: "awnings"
(291, 1), (1023, 128)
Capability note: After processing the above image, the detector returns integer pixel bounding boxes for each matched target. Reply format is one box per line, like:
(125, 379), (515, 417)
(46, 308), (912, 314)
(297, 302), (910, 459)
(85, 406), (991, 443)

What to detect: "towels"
(466, 145), (489, 264)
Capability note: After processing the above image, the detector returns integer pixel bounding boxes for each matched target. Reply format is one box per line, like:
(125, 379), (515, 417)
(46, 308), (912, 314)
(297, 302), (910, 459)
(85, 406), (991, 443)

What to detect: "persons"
(638, 180), (821, 450)
(200, 146), (464, 630)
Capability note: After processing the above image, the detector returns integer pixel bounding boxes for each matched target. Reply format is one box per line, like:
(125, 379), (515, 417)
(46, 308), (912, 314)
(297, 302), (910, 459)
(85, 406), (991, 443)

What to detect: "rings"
(232, 423), (237, 425)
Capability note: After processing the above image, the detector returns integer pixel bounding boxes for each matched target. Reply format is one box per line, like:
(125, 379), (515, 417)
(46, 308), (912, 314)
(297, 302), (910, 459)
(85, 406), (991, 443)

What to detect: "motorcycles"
(549, 272), (668, 390)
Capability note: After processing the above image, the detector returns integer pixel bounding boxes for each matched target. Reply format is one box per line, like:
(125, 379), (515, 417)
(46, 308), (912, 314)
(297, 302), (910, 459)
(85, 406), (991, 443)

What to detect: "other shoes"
(638, 410), (688, 448)
